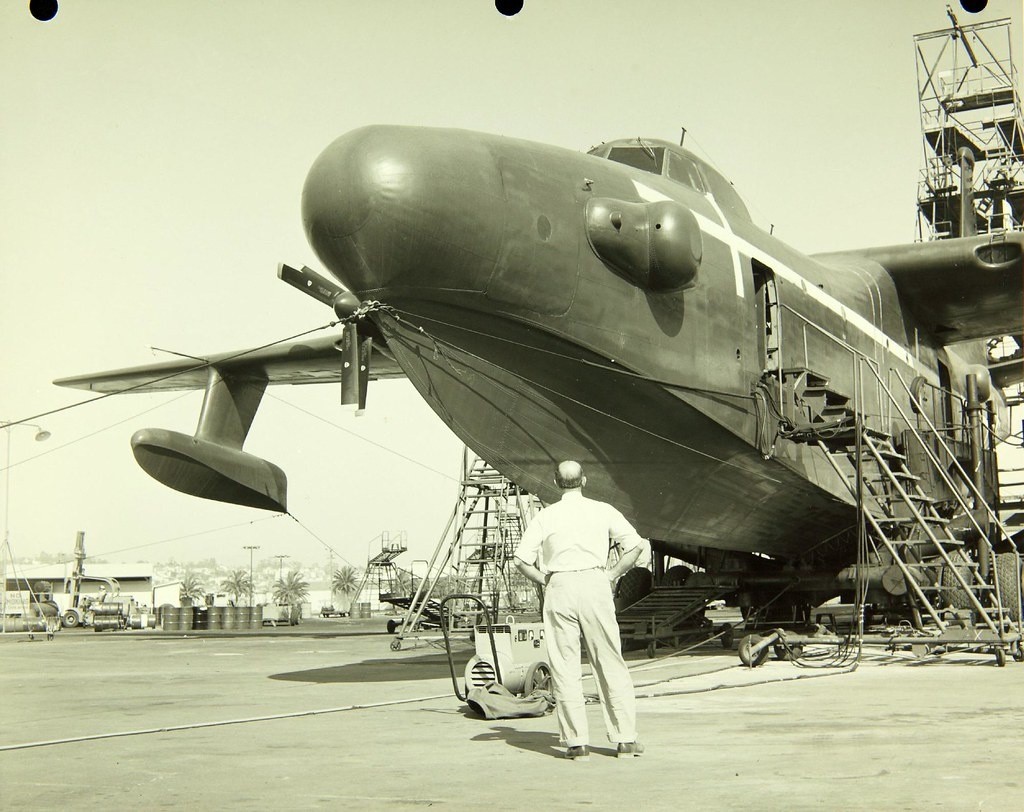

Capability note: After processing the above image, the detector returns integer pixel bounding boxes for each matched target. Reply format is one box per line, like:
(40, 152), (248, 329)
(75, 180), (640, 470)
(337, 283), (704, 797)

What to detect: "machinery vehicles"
(55, 530), (134, 628)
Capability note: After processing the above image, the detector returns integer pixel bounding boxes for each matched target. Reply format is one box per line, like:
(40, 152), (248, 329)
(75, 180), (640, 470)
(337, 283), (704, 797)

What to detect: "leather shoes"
(565, 745), (589, 762)
(617, 741), (645, 758)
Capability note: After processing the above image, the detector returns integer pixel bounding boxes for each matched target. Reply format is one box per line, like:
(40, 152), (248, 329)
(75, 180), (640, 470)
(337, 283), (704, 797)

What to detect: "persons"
(514, 460), (645, 760)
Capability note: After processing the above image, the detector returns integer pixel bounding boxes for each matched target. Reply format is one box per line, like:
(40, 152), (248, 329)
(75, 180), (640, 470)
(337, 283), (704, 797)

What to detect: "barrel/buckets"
(192, 607), (207, 630)
(361, 603), (371, 618)
(178, 608), (193, 630)
(163, 608), (178, 629)
(207, 607), (221, 630)
(221, 607), (235, 628)
(249, 607), (262, 628)
(350, 603), (360, 618)
(236, 607), (250, 629)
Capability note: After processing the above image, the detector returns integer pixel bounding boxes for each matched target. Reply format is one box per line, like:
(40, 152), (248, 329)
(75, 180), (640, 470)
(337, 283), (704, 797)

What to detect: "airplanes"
(52, 122), (1024, 635)
